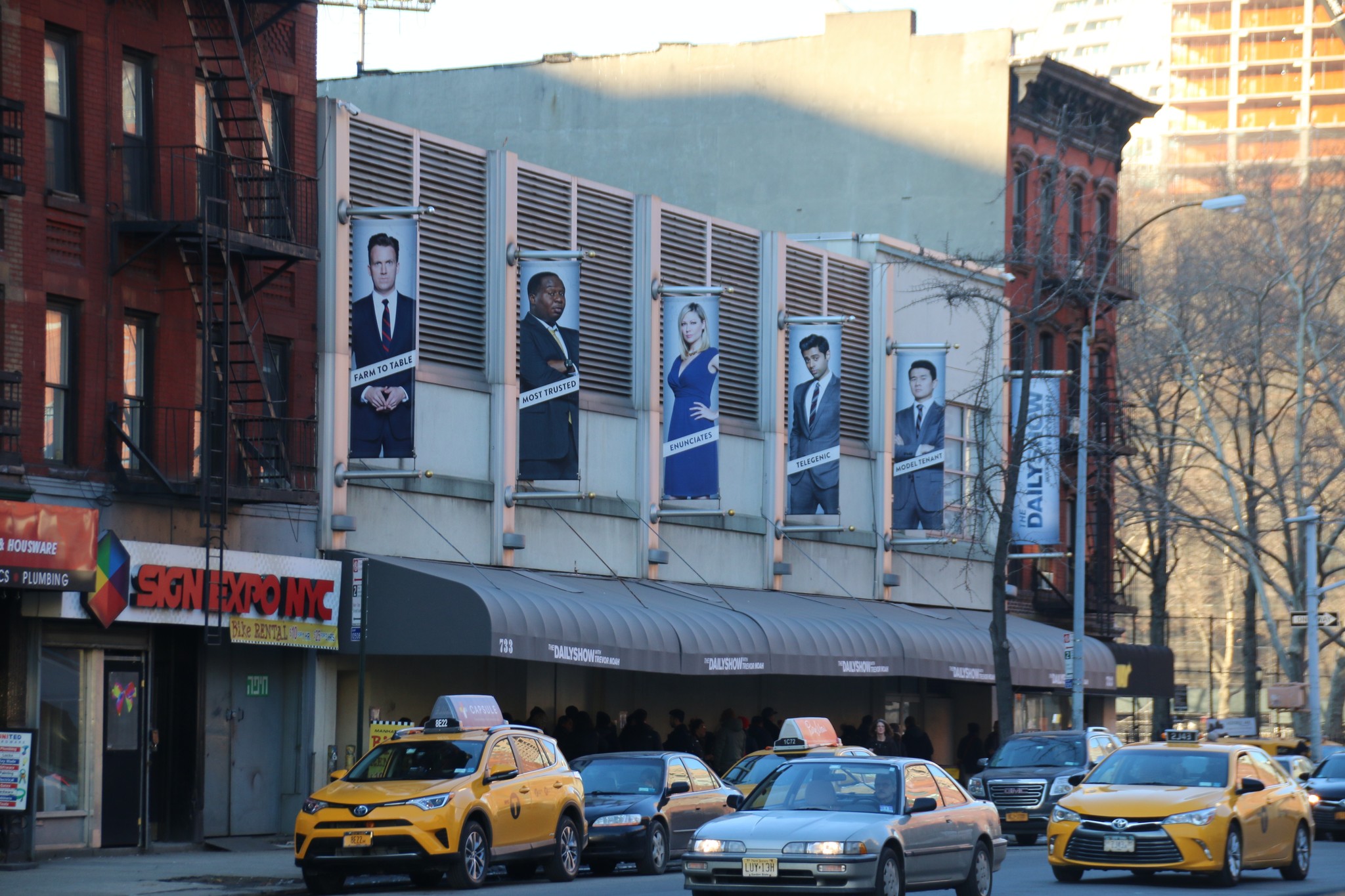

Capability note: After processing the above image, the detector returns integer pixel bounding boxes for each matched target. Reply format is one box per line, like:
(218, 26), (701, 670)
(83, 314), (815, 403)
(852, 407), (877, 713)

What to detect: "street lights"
(1067, 191), (1249, 733)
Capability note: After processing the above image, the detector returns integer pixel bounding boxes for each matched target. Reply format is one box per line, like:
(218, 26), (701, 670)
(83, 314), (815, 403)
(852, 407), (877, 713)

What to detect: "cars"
(565, 750), (745, 878)
(1113, 710), (1345, 842)
(718, 717), (879, 810)
(680, 757), (1009, 896)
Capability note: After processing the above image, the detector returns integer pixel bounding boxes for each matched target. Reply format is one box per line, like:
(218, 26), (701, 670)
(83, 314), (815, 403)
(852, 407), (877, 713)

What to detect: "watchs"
(563, 358), (573, 373)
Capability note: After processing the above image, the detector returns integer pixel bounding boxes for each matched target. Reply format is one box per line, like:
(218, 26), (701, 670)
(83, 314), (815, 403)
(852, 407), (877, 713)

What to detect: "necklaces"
(686, 347), (701, 356)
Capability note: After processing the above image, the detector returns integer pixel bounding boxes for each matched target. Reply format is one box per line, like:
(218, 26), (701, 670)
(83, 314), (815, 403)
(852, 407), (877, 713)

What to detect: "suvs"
(293, 694), (591, 896)
(967, 725), (1124, 847)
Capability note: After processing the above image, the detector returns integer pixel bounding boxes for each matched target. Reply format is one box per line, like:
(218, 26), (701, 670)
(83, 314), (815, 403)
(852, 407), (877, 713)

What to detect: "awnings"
(342, 545), (1118, 694)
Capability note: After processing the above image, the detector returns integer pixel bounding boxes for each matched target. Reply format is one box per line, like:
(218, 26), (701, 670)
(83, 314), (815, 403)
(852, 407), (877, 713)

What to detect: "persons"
(666, 304), (719, 499)
(393, 702), (1004, 787)
(514, 271), (579, 484)
(893, 356), (946, 532)
(350, 231), (418, 463)
(783, 332), (840, 517)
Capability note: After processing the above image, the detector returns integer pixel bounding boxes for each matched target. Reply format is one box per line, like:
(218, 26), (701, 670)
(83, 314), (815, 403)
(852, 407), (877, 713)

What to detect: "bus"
(1046, 729), (1317, 889)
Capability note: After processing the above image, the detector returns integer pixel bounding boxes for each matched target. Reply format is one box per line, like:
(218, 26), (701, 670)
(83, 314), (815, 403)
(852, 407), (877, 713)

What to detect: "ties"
(809, 382), (821, 431)
(915, 404), (924, 438)
(382, 299), (392, 353)
(548, 328), (571, 423)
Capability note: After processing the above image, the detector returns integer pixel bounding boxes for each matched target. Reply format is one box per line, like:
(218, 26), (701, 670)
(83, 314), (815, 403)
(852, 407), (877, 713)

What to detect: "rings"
(699, 411), (701, 414)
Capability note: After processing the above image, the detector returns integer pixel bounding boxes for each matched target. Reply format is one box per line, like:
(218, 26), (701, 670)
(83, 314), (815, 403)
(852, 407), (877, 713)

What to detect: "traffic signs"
(1290, 610), (1341, 627)
